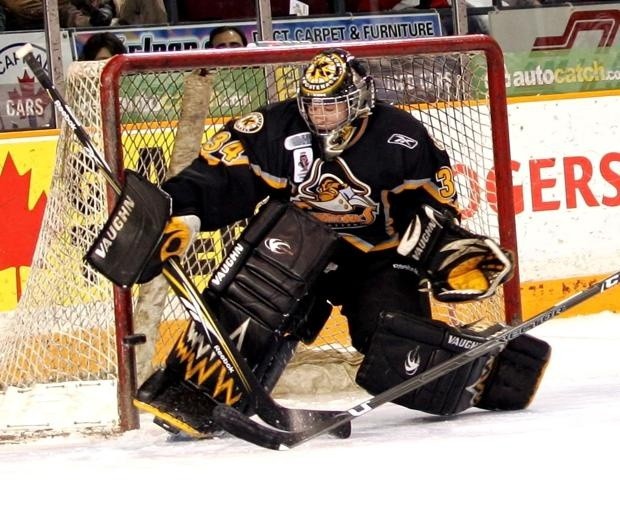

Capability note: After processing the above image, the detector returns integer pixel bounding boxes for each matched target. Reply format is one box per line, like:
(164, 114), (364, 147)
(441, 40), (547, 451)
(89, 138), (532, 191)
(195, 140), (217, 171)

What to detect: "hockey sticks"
(212, 272), (619, 451)
(15, 42), (351, 439)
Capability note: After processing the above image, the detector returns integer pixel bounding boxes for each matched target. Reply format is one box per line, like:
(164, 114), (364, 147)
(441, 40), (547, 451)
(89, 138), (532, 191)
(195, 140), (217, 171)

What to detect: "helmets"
(296, 49), (376, 137)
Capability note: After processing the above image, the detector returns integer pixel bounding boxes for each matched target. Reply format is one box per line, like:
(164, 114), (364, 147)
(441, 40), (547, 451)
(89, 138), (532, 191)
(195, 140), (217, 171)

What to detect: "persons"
(207, 27), (249, 49)
(0, 1), (171, 29)
(83, 31), (127, 61)
(92, 44), (550, 432)
